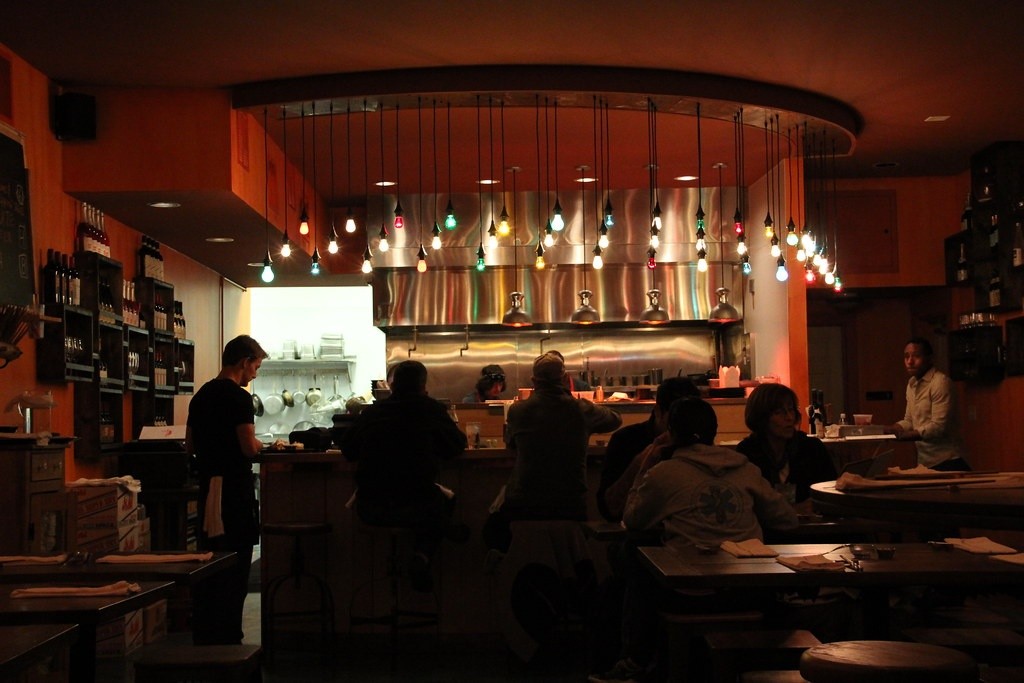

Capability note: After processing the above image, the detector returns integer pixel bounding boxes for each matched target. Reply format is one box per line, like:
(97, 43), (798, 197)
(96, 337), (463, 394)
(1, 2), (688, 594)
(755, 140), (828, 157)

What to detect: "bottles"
(955, 164), (1024, 309)
(808, 387), (825, 437)
(596, 385), (604, 402)
(958, 341), (978, 376)
(838, 413), (846, 425)
(44, 202), (187, 446)
(449, 405), (459, 428)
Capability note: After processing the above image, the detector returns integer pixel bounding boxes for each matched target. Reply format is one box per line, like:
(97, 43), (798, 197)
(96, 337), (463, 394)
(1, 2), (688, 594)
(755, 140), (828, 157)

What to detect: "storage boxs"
(66, 485), (167, 659)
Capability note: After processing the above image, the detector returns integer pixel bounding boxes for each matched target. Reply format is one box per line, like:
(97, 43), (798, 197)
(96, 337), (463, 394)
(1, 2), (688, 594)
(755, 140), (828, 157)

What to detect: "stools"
(665, 589), (1024, 683)
(135, 645), (265, 683)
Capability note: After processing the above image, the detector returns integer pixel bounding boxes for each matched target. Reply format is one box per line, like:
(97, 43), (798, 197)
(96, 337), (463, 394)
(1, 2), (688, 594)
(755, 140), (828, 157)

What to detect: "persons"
(186, 336), (261, 644)
(587, 397), (800, 683)
(882, 337), (966, 471)
(339, 362), (472, 590)
(597, 376), (704, 657)
(462, 365), (507, 404)
(733, 384), (839, 503)
(482, 354), (621, 556)
(523, 351), (592, 391)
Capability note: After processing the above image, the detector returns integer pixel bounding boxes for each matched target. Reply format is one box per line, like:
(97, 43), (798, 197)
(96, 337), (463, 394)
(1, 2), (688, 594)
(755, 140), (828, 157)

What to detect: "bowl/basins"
(370, 380), (385, 400)
(518, 388), (535, 400)
(570, 391), (595, 401)
(853, 414), (872, 425)
(0, 425), (19, 433)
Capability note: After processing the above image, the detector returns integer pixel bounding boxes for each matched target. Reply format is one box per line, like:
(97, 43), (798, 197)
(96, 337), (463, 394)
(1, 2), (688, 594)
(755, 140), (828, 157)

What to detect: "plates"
(708, 387), (746, 398)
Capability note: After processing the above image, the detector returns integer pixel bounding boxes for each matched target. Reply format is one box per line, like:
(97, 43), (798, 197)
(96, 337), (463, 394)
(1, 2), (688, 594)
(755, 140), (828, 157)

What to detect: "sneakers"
(589, 658), (640, 683)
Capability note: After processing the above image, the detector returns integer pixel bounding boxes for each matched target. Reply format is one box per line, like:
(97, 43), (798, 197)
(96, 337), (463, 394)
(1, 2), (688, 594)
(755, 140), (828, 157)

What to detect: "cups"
(636, 388), (650, 399)
(707, 379), (719, 388)
(959, 312), (999, 329)
(744, 387), (755, 398)
(465, 422), (481, 450)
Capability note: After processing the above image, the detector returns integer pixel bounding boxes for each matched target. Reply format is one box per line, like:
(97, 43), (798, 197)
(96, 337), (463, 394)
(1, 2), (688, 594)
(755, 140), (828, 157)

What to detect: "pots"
(251, 373), (366, 441)
(687, 368), (719, 386)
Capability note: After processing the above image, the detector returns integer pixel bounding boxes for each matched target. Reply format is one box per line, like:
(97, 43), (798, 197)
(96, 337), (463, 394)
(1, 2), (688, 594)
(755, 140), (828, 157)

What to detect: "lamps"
(503, 165), (534, 327)
(708, 163), (738, 323)
(640, 164), (671, 324)
(571, 166), (602, 326)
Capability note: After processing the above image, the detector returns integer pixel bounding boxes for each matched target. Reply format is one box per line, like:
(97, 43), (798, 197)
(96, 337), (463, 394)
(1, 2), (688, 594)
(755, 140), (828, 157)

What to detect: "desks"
(0, 552), (239, 683)
(582, 475), (1024, 588)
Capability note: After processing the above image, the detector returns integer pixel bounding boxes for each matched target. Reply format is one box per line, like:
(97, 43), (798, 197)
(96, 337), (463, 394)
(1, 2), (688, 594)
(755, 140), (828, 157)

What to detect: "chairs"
(264, 521), (600, 680)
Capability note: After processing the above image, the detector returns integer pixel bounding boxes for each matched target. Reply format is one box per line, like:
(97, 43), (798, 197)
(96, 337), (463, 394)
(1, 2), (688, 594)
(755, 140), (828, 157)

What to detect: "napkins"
(720, 460), (1024, 572)
(0, 554), (67, 566)
(96, 552), (214, 564)
(10, 579), (140, 596)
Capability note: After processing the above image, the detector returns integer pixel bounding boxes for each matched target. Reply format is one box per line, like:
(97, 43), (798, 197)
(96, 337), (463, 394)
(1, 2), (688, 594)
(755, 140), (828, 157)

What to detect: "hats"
(482, 365), (506, 380)
(532, 351), (566, 379)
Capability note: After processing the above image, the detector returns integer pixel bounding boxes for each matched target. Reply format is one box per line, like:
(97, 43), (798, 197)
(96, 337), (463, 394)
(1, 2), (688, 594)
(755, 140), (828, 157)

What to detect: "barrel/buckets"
(579, 368), (662, 385)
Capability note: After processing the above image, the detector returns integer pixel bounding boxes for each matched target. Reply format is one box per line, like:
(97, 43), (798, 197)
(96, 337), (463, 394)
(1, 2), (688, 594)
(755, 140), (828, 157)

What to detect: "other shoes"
(446, 522), (471, 544)
(409, 554), (434, 594)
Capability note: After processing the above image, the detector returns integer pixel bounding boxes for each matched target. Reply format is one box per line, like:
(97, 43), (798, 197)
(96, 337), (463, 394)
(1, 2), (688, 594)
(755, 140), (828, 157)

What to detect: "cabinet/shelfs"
(0, 426), (82, 555)
(943, 141), (1024, 379)
(138, 484), (199, 634)
(37, 252), (195, 459)
